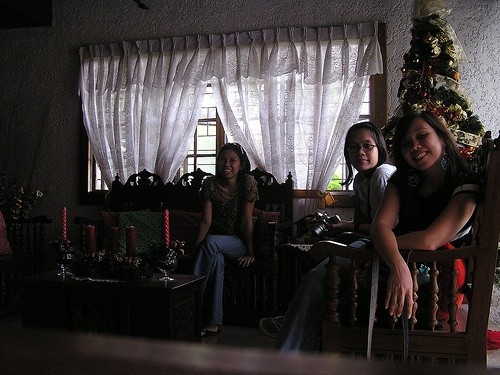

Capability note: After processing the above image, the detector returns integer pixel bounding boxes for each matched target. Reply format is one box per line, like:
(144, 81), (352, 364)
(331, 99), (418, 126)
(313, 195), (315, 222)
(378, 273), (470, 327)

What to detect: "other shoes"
(259, 315), (284, 338)
(202, 327), (220, 336)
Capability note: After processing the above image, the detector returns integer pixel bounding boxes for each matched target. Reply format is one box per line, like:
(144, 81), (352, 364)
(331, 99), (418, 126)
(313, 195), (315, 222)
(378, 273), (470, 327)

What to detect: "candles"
(126, 226), (135, 257)
(164, 209), (169, 251)
(87, 225), (96, 253)
(111, 227), (118, 249)
(60, 206), (67, 241)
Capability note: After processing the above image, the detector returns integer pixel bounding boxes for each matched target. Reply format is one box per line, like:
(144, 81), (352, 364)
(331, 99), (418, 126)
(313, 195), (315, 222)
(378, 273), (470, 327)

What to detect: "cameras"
(310, 213), (341, 237)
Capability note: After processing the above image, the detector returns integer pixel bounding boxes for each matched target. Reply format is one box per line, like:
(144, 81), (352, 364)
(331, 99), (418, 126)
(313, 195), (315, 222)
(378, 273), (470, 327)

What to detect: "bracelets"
(354, 222), (360, 232)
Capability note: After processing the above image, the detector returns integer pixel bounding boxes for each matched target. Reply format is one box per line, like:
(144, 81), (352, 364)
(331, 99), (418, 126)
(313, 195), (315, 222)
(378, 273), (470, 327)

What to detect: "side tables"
(293, 238), (337, 296)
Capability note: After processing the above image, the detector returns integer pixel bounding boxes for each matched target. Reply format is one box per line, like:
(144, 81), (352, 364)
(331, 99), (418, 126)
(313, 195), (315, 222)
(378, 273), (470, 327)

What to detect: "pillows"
(100, 207), (280, 252)
(0, 212), (12, 255)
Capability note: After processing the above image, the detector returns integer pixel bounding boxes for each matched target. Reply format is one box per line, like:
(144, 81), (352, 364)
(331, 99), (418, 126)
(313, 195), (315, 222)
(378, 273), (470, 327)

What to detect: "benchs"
(74, 168), (295, 326)
(309, 131), (500, 371)
(0, 216), (53, 319)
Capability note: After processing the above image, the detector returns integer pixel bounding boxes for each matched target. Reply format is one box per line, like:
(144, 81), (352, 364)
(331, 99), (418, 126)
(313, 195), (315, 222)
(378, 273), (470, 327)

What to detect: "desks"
(22, 271), (205, 343)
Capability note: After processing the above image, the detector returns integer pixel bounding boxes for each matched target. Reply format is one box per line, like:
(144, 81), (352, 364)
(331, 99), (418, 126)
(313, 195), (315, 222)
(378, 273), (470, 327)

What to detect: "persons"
(260, 122), (398, 337)
(275, 110), (482, 361)
(191, 143), (260, 336)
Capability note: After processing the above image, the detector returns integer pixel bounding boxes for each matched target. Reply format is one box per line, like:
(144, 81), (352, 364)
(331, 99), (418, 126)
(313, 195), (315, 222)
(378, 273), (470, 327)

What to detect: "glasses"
(346, 143), (379, 154)
